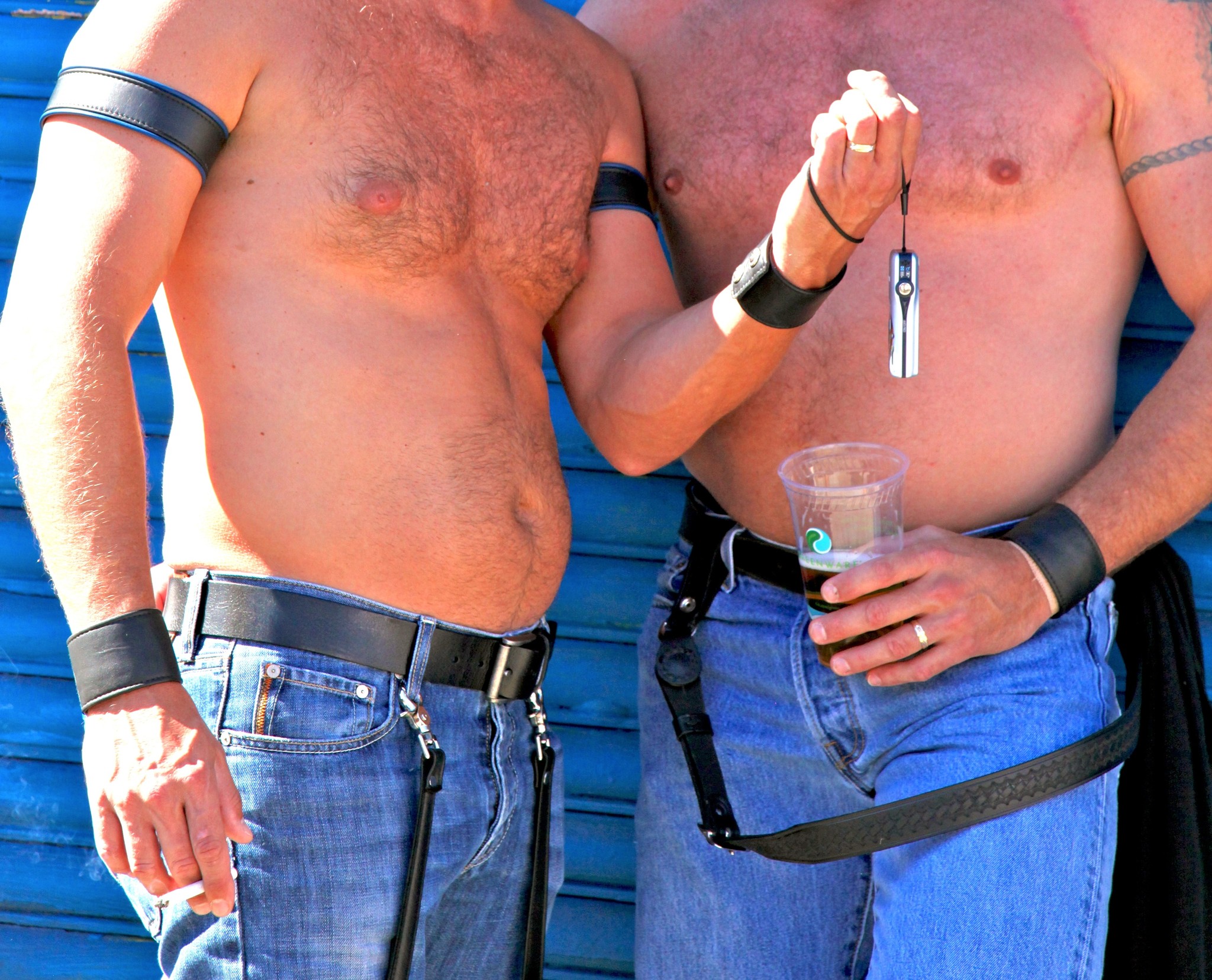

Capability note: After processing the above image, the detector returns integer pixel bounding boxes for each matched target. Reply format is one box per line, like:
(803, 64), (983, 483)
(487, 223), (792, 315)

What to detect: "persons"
(0, 0), (920, 980)
(575, 0), (1212, 979)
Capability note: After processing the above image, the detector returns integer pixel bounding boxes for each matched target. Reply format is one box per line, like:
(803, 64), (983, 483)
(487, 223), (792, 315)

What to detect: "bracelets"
(998, 501), (1106, 620)
(730, 231), (847, 328)
(66, 609), (183, 714)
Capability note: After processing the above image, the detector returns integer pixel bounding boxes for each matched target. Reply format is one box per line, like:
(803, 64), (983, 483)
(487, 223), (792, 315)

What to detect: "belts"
(680, 481), (1022, 597)
(161, 578), (557, 697)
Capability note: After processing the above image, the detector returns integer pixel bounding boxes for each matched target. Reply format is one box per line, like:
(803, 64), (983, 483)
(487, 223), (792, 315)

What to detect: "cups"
(777, 442), (910, 668)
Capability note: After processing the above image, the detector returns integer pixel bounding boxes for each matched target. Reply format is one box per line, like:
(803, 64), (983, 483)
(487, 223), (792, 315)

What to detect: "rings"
(910, 619), (929, 650)
(846, 140), (876, 153)
